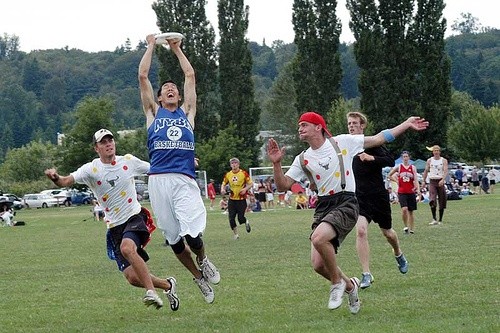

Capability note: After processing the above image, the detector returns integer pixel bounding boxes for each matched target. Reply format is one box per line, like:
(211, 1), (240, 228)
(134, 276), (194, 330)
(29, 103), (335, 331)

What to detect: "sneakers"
(162, 276), (180, 311)
(142, 289), (163, 310)
(233, 235), (240, 240)
(196, 255), (221, 284)
(246, 220), (251, 233)
(429, 220), (438, 225)
(409, 229), (414, 233)
(348, 276), (360, 313)
(328, 278), (347, 310)
(394, 254), (408, 274)
(193, 269), (215, 303)
(438, 220), (442, 225)
(360, 272), (376, 289)
(403, 225), (409, 233)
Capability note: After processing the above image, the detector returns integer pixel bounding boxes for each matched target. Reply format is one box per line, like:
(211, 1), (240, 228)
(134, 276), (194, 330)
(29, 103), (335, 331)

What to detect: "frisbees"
(151, 31), (184, 44)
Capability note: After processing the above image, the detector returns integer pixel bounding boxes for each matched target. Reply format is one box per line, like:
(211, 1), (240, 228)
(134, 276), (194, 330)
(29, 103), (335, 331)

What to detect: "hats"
(93, 127), (114, 143)
(298, 111), (332, 137)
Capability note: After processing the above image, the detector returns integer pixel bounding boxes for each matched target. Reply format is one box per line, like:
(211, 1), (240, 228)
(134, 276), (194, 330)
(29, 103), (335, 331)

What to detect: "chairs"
(93, 205), (103, 221)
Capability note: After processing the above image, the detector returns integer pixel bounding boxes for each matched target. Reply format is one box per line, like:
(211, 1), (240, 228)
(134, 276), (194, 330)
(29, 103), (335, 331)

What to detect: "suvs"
(40, 189), (62, 194)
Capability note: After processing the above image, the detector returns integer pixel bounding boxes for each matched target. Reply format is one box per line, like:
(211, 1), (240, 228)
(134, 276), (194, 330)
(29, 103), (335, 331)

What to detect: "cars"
(20, 193), (53, 209)
(53, 189), (93, 207)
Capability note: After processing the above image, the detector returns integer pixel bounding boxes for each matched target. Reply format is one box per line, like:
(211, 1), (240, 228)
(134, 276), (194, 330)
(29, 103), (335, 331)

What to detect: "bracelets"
(382, 129), (395, 143)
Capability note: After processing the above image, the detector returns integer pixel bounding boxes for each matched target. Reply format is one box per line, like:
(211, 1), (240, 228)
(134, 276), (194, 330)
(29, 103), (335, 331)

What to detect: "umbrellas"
(411, 159), (427, 172)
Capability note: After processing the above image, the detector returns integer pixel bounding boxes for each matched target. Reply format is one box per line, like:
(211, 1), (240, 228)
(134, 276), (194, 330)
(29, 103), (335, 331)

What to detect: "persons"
(66, 187), (74, 209)
(245, 196), (261, 212)
(220, 158), (254, 240)
(489, 167), (496, 195)
(471, 165), (480, 194)
(253, 178), (259, 201)
(455, 166), (463, 186)
(266, 176), (275, 208)
(295, 193), (307, 212)
(421, 145), (448, 226)
(43, 129), (179, 310)
(220, 194), (230, 212)
(259, 181), (267, 212)
(137, 31), (221, 306)
(207, 179), (217, 212)
(278, 191), (293, 209)
(1, 205), (25, 227)
(388, 150), (420, 233)
(92, 198), (104, 222)
(346, 109), (409, 289)
(308, 190), (319, 209)
(269, 112), (429, 315)
(479, 173), (490, 195)
(387, 186), (398, 202)
(462, 166), (468, 189)
